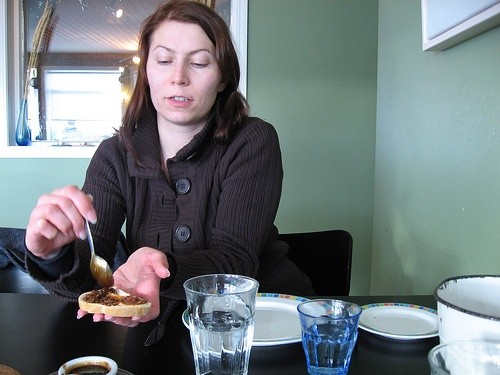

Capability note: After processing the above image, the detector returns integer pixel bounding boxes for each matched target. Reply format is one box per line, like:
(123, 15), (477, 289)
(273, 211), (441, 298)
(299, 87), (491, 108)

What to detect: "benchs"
(0, 228), (353, 296)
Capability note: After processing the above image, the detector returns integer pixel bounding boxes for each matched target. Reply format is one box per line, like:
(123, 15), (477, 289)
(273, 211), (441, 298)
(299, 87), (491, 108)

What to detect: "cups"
(433, 274), (500, 348)
(179, 273), (260, 375)
(296, 299), (363, 375)
(427, 338), (500, 375)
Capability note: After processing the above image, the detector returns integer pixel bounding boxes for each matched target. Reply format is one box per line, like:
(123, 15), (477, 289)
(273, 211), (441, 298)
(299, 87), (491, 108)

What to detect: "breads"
(78, 287), (152, 316)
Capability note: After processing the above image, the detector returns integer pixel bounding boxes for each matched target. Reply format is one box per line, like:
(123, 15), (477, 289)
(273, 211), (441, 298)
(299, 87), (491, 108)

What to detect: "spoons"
(84, 220), (114, 287)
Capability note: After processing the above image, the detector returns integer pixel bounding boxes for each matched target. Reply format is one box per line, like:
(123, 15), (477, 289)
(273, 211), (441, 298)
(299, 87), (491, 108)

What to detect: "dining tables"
(0, 294), (440, 375)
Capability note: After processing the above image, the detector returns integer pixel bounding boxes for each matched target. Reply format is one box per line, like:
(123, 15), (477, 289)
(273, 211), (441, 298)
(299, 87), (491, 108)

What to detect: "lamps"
(104, 0), (124, 19)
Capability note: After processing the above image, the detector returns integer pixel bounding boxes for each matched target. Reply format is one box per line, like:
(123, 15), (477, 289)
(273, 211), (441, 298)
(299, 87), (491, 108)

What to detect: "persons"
(22, 0), (317, 328)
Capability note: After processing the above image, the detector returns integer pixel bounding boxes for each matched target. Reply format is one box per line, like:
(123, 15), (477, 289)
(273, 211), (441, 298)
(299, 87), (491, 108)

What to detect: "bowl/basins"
(58, 355), (119, 375)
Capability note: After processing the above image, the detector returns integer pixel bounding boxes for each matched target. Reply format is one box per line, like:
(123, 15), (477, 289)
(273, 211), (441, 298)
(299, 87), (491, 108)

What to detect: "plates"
(181, 292), (338, 347)
(347, 302), (440, 341)
